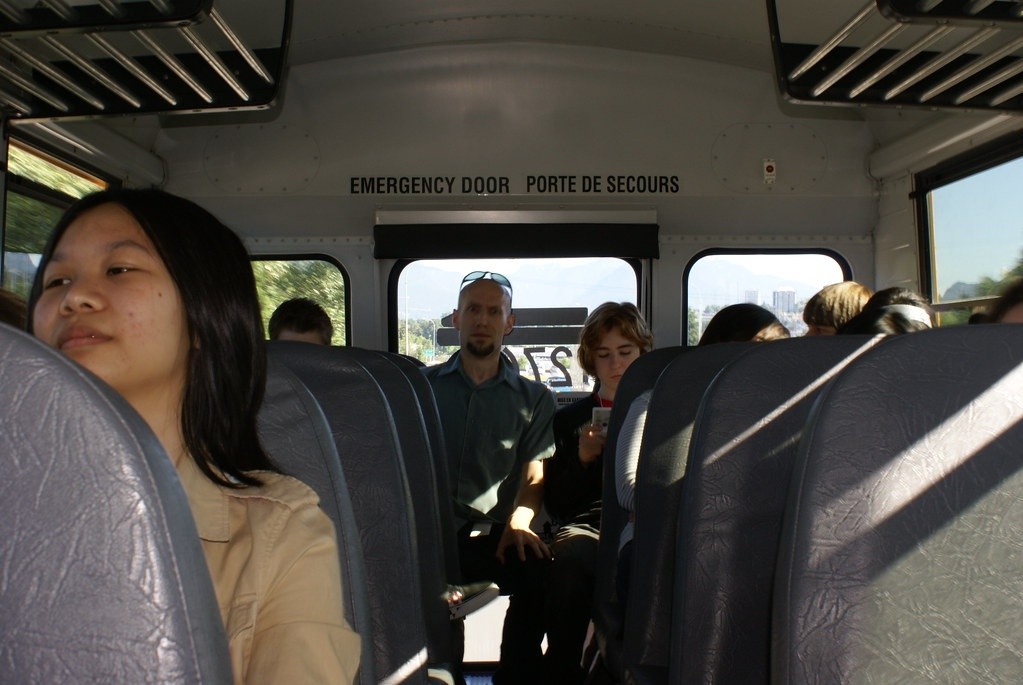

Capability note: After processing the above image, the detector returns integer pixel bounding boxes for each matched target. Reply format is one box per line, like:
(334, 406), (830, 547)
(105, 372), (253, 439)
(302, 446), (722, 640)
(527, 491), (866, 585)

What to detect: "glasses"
(459, 271), (514, 307)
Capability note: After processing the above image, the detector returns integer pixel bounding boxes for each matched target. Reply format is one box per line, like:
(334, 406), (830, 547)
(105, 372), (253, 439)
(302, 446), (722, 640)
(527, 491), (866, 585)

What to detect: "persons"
(420, 269), (935, 685)
(25, 190), (364, 685)
(268, 297), (333, 345)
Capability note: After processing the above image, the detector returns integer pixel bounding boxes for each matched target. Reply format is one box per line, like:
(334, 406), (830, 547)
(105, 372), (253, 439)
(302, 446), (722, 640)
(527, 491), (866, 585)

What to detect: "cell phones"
(593, 407), (611, 438)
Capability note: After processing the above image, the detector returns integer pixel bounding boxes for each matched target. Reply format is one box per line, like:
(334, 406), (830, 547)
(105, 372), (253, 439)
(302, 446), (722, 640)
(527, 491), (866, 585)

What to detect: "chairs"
(552, 322), (1023, 685)
(0, 322), (458, 685)
